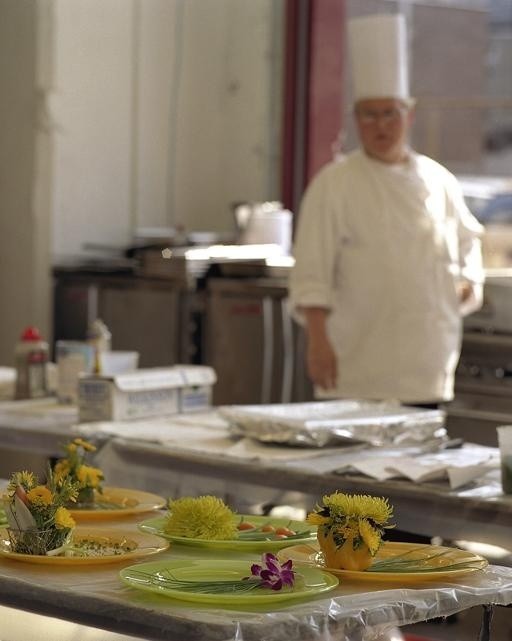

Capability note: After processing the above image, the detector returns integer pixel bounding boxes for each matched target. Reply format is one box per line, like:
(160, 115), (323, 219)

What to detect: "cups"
(496, 424), (511, 493)
(99, 351), (140, 376)
(53, 339), (96, 407)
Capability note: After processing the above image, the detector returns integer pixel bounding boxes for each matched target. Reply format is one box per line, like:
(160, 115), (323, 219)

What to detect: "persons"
(284, 93), (487, 410)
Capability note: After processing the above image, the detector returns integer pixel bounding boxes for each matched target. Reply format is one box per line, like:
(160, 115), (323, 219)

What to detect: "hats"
(347, 14), (409, 104)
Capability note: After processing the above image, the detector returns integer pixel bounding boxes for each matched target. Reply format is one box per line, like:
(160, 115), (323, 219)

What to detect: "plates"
(117, 559), (341, 608)
(66, 485), (169, 518)
(0, 528), (169, 568)
(139, 513), (314, 553)
(276, 543), (487, 584)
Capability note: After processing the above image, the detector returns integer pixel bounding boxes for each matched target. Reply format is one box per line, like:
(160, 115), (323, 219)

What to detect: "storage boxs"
(77, 364), (218, 421)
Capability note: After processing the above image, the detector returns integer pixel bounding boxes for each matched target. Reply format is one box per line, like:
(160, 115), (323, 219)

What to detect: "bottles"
(86, 316), (112, 377)
(14, 325), (50, 399)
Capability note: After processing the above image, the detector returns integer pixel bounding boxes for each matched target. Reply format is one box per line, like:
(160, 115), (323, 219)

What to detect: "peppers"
(317, 526), (373, 571)
